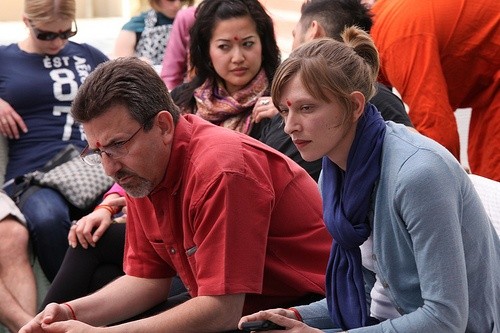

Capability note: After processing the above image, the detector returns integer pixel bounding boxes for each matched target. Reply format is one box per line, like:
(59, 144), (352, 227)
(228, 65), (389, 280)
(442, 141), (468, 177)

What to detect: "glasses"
(28, 19), (78, 41)
(81, 109), (170, 163)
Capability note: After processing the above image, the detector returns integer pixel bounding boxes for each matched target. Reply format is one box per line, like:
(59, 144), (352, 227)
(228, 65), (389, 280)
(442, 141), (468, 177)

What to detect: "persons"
(0, 0), (500, 333)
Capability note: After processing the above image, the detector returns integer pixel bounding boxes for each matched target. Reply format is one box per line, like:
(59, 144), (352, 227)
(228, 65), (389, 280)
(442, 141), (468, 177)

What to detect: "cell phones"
(241, 322), (286, 331)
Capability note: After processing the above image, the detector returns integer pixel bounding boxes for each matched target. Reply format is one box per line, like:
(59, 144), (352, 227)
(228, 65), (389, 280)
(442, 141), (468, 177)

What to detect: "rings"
(72, 220), (77, 225)
(260, 100), (269, 105)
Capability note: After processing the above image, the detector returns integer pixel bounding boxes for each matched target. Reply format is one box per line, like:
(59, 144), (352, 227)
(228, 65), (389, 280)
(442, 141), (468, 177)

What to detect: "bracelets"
(62, 303), (76, 320)
(289, 308), (303, 322)
(93, 204), (115, 214)
